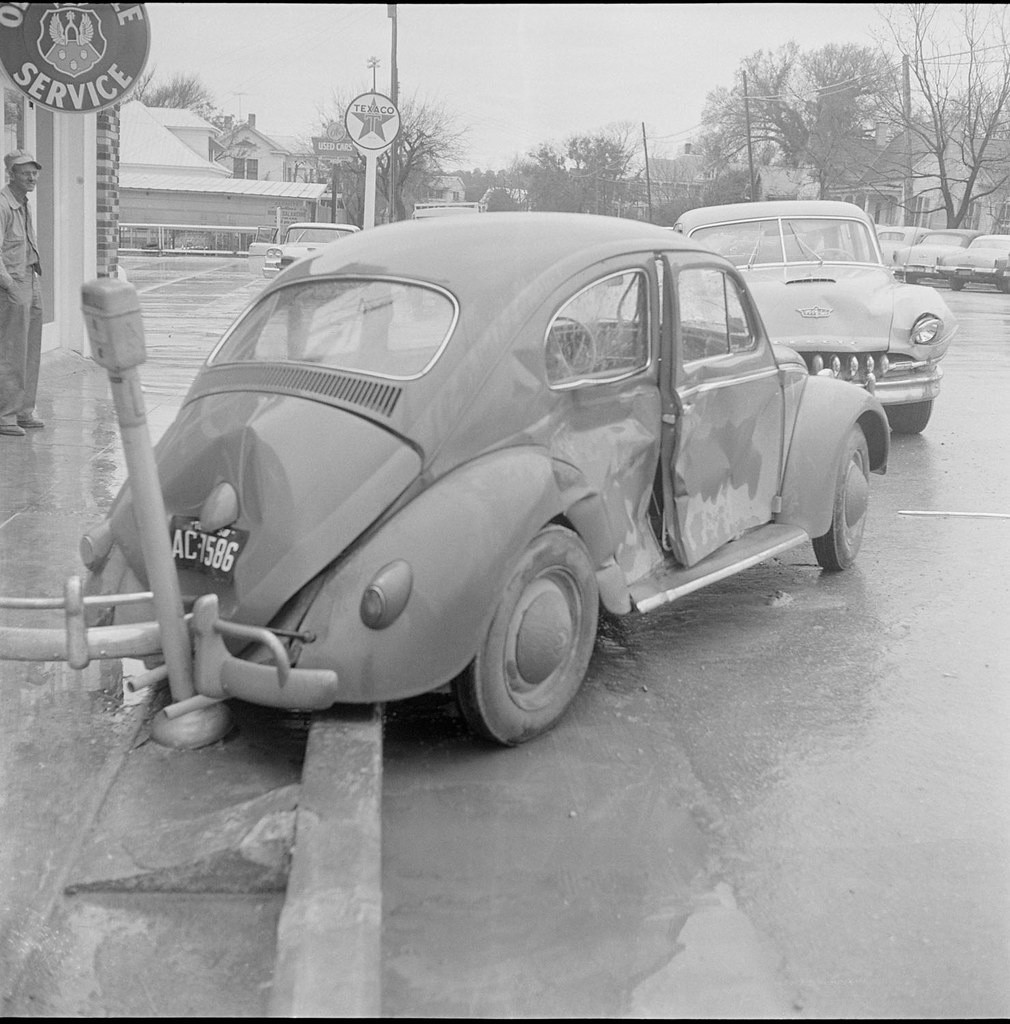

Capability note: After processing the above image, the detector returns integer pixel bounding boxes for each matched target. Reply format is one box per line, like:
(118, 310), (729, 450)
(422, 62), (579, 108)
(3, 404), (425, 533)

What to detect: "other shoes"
(0, 425), (24, 436)
(18, 416), (44, 427)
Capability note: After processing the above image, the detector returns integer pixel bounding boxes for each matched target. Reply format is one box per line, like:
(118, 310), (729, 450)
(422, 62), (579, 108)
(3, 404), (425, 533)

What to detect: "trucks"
(410, 201), (484, 219)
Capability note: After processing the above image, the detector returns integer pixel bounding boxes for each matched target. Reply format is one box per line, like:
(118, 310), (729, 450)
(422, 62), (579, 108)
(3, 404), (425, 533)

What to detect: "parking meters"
(79, 277), (228, 750)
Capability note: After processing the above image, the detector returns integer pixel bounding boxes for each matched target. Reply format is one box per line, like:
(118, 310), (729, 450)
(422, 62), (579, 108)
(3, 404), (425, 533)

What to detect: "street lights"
(368, 56), (382, 92)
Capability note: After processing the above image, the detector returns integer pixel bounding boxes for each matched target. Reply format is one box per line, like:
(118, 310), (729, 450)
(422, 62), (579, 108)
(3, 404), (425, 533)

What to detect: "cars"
(262, 222), (362, 281)
(0, 212), (889, 748)
(664, 201), (958, 433)
(873, 224), (1010, 294)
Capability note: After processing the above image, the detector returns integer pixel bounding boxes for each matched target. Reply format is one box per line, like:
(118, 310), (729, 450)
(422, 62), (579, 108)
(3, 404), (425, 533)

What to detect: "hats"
(3, 149), (42, 173)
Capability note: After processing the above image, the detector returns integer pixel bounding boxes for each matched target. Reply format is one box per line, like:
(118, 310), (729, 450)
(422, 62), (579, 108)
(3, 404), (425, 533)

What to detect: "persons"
(0, 148), (44, 436)
